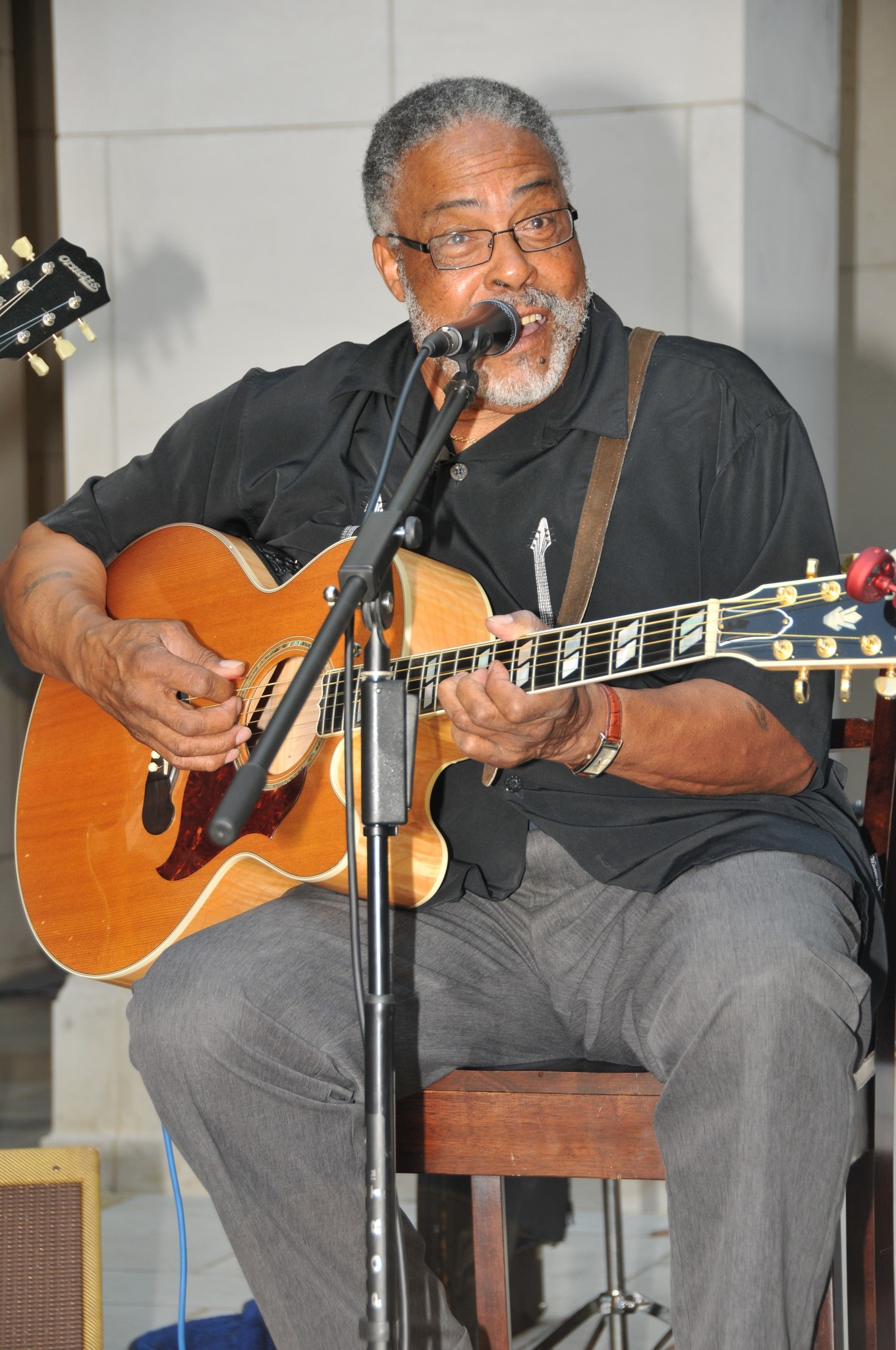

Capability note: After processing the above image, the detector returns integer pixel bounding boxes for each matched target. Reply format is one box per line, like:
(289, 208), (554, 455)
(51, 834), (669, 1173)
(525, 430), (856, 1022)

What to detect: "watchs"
(564, 682), (625, 779)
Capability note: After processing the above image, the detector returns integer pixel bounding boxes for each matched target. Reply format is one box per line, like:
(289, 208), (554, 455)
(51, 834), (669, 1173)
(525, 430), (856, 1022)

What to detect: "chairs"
(396, 658), (896, 1350)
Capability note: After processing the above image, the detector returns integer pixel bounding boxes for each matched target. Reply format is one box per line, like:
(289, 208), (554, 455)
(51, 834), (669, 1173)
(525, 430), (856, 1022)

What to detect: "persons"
(0, 75), (887, 1350)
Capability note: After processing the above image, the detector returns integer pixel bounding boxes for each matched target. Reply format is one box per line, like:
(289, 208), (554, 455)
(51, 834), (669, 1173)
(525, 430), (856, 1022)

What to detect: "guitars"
(14, 521), (896, 990)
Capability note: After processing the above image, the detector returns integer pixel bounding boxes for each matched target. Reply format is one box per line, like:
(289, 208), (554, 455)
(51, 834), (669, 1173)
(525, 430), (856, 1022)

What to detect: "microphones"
(423, 299), (523, 358)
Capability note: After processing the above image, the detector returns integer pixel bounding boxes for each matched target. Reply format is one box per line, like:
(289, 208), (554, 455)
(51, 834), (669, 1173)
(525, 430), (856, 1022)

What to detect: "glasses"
(387, 202), (578, 270)
